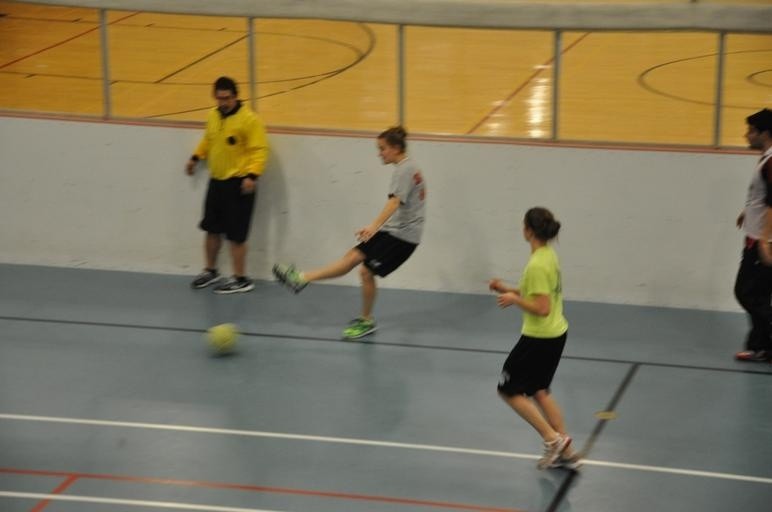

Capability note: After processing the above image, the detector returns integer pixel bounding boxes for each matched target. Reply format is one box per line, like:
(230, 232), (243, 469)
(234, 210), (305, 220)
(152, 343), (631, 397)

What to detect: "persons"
(271, 127), (427, 341)
(184, 74), (270, 295)
(488, 207), (584, 471)
(733, 108), (772, 361)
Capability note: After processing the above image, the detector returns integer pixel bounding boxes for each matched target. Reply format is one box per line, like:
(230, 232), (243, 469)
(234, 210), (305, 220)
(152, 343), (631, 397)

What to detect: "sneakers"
(189, 267), (221, 289)
(342, 314), (378, 340)
(534, 434), (572, 471)
(735, 348), (771, 363)
(548, 449), (585, 471)
(211, 274), (255, 295)
(271, 262), (308, 294)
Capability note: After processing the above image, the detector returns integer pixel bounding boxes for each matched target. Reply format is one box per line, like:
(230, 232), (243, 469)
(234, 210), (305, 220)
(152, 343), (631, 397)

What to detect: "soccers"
(205, 324), (237, 354)
